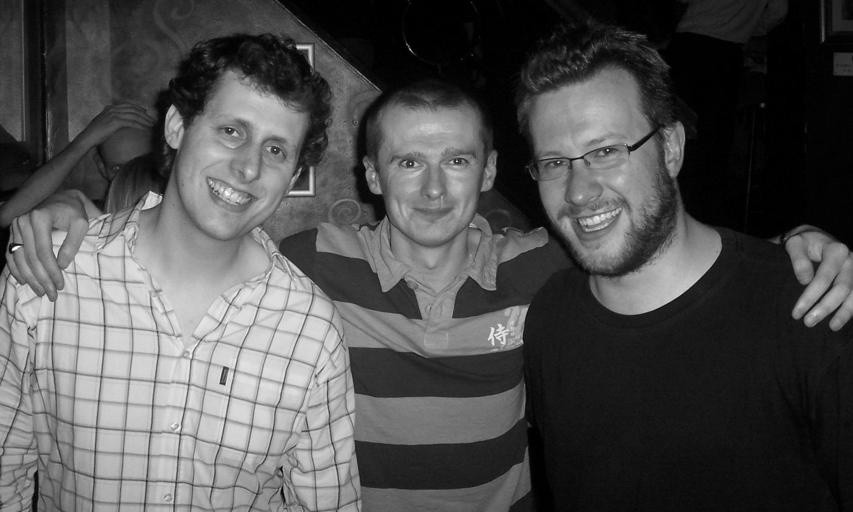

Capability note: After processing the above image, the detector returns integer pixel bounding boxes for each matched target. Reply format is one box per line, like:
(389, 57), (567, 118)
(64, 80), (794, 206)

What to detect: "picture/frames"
(277, 41), (319, 200)
(814, 0), (850, 47)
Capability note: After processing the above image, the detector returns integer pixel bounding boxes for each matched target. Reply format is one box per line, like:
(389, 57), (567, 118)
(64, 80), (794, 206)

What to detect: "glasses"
(524, 126), (661, 183)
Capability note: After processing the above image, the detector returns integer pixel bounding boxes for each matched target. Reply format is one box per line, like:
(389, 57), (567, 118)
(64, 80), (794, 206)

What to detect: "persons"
(5, 82), (853, 512)
(0, 32), (363, 512)
(0, 100), (169, 279)
(517, 20), (852, 512)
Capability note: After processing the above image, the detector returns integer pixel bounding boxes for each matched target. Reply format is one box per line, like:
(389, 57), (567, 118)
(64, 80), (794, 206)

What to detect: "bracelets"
(779, 230), (791, 247)
